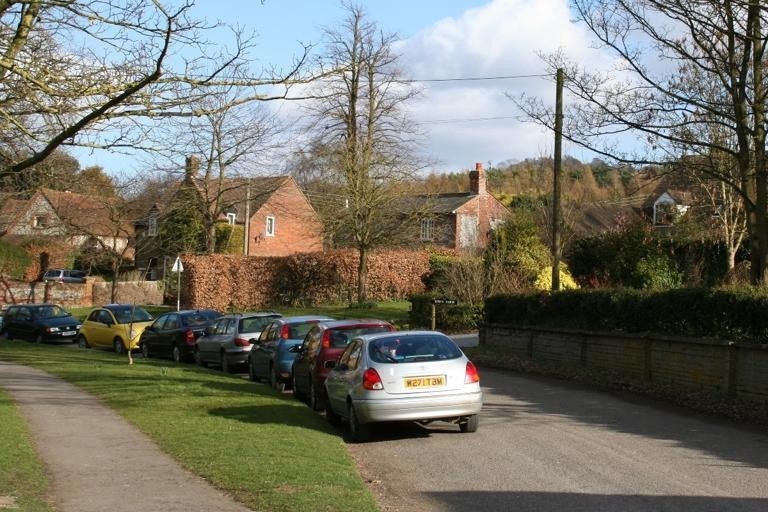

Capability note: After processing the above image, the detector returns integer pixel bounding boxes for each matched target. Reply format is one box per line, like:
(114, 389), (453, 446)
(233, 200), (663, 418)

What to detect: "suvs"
(41, 268), (87, 283)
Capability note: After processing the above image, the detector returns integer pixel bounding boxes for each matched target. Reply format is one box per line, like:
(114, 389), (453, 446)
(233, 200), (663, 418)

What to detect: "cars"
(245, 313), (485, 444)
(0, 302), (284, 375)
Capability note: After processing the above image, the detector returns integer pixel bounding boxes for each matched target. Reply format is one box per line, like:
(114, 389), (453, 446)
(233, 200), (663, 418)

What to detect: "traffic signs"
(432, 299), (458, 305)
